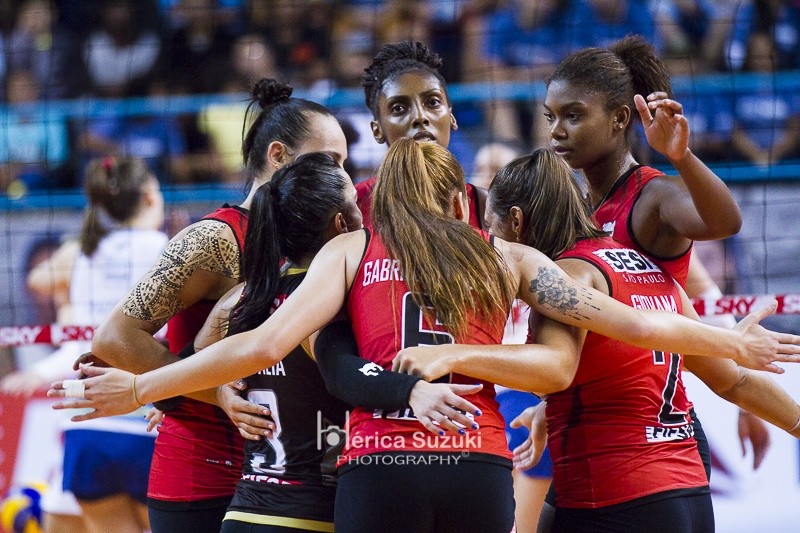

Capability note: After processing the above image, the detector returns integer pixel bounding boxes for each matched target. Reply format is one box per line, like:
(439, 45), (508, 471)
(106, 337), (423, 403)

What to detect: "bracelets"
(133, 374), (147, 408)
(786, 420), (800, 432)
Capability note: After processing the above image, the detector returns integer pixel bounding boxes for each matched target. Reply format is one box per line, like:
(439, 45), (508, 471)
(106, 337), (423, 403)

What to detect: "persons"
(0, 0), (800, 533)
